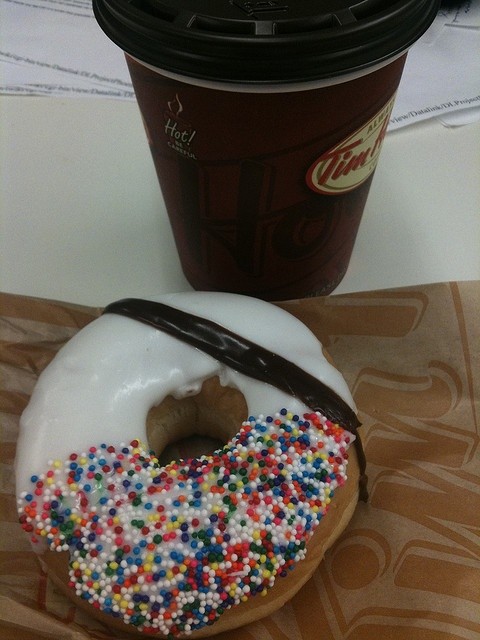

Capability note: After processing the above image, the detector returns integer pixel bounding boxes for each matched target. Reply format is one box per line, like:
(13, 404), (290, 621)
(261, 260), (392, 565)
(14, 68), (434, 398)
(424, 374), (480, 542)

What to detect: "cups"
(91, 0), (440, 299)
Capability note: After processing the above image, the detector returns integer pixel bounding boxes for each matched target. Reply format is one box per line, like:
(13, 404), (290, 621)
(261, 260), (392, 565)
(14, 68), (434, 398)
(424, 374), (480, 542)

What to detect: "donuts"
(14, 292), (366, 639)
